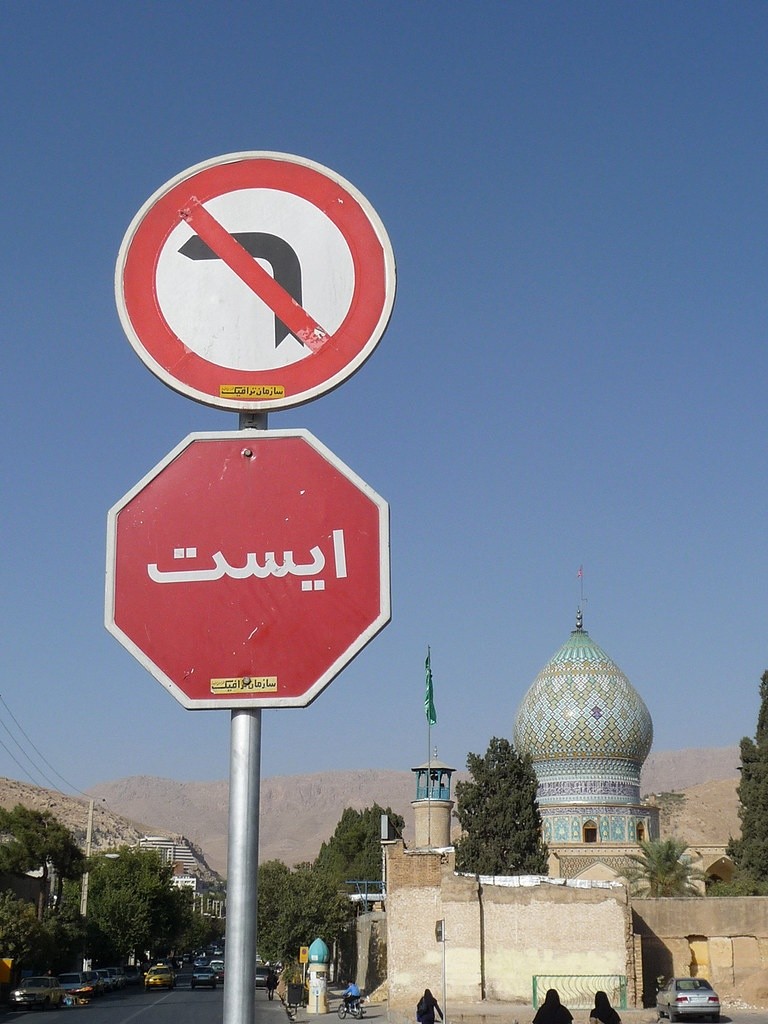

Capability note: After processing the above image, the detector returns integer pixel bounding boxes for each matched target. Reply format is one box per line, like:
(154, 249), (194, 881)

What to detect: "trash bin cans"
(287, 983), (303, 1012)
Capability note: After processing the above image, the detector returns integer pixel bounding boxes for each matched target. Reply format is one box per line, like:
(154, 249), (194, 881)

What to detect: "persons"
(531, 989), (574, 1024)
(589, 991), (622, 1024)
(343, 981), (361, 1013)
(417, 989), (443, 1024)
(266, 970), (275, 1001)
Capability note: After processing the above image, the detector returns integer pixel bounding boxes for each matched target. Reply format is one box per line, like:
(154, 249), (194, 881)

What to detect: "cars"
(193, 956), (209, 967)
(209, 960), (224, 984)
(655, 977), (721, 1024)
(144, 963), (175, 992)
(93, 968), (114, 992)
(122, 965), (141, 985)
(190, 965), (217, 990)
(172, 944), (224, 966)
(104, 966), (129, 990)
(7, 976), (66, 1012)
(255, 966), (275, 988)
(83, 971), (104, 1000)
(56, 971), (91, 1004)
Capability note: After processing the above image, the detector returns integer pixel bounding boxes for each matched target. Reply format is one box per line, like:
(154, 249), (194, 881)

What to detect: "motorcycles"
(337, 994), (367, 1020)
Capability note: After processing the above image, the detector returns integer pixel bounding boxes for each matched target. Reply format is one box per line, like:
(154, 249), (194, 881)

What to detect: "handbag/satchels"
(419, 997), (428, 1016)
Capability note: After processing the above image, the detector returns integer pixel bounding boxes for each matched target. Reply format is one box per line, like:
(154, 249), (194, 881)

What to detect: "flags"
(577, 568), (583, 578)
(425, 654), (439, 724)
